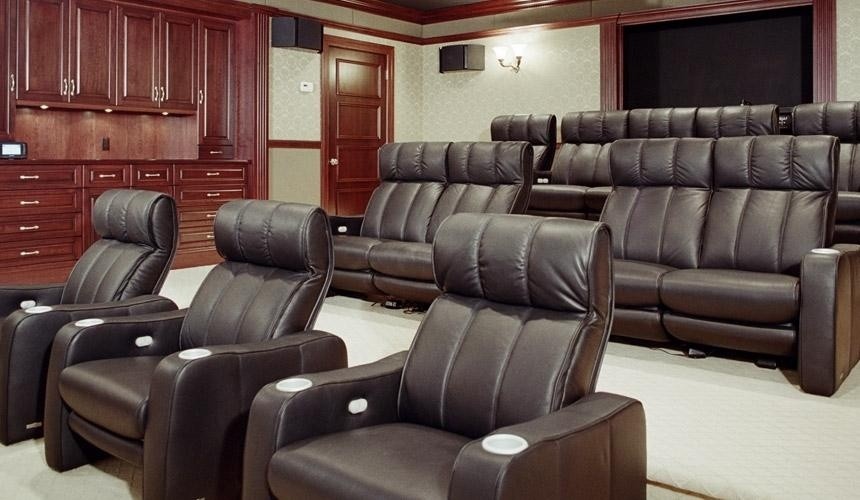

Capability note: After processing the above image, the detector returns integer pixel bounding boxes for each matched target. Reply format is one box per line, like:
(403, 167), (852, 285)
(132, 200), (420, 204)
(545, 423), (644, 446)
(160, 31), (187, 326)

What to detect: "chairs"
(0, 187), (180, 446)
(491, 113), (557, 170)
(791, 100), (859, 243)
(241, 212), (647, 500)
(44, 199), (347, 499)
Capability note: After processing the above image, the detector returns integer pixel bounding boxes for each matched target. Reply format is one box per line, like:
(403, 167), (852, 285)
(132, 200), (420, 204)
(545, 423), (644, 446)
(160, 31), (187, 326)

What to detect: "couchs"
(599, 135), (860, 397)
(529, 104), (780, 221)
(326, 142), (533, 304)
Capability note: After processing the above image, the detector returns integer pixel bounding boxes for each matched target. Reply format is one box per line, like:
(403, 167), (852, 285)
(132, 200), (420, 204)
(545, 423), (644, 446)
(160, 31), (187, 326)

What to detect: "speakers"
(271, 15), (324, 54)
(438, 45), (486, 74)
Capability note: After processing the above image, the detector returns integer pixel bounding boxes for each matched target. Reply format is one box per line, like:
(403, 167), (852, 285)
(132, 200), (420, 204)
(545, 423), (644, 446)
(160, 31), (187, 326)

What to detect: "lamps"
(490, 44), (528, 74)
